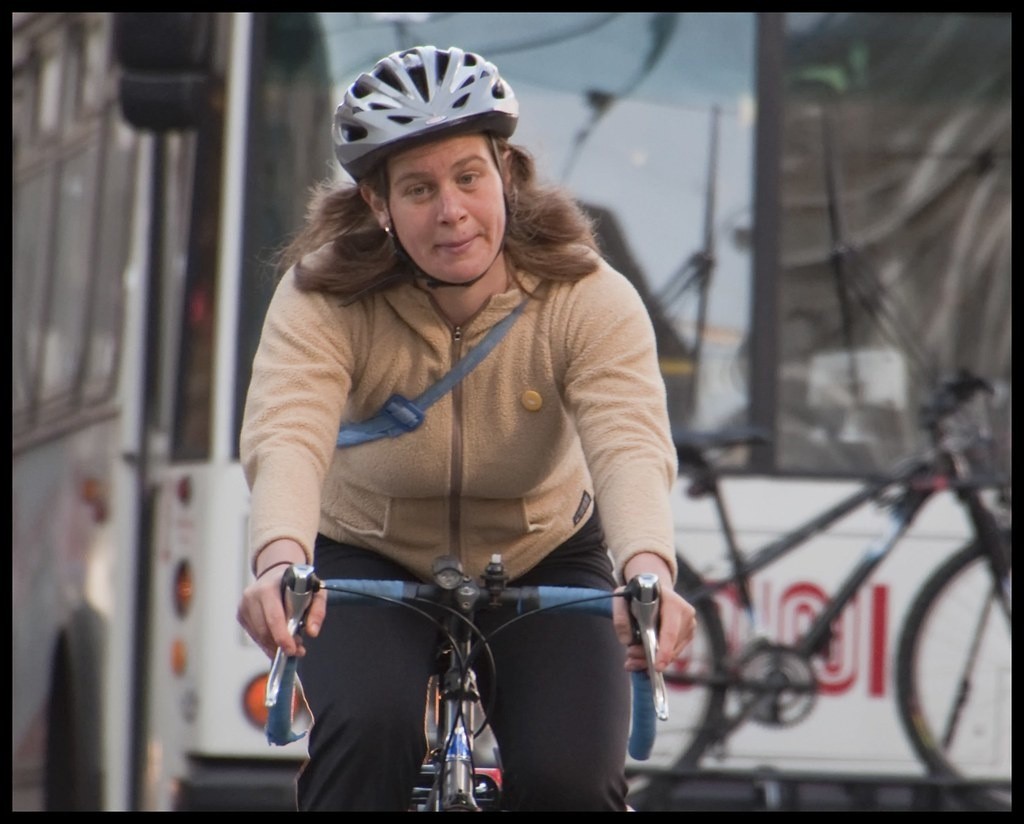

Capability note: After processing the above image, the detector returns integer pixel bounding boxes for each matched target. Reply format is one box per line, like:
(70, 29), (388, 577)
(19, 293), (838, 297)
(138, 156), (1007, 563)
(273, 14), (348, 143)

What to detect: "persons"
(236, 45), (699, 812)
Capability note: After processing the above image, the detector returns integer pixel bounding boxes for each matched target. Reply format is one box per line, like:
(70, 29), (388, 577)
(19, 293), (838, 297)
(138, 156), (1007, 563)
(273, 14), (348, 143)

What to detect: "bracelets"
(256, 562), (294, 579)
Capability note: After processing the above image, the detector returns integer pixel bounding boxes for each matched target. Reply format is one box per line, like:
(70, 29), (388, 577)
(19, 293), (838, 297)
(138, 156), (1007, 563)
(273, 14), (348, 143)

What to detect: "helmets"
(330, 45), (520, 183)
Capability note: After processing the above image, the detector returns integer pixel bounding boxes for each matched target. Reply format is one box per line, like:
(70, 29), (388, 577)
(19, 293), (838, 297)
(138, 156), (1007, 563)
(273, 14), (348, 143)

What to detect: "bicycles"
(261, 561), (672, 812)
(613, 363), (1015, 789)
